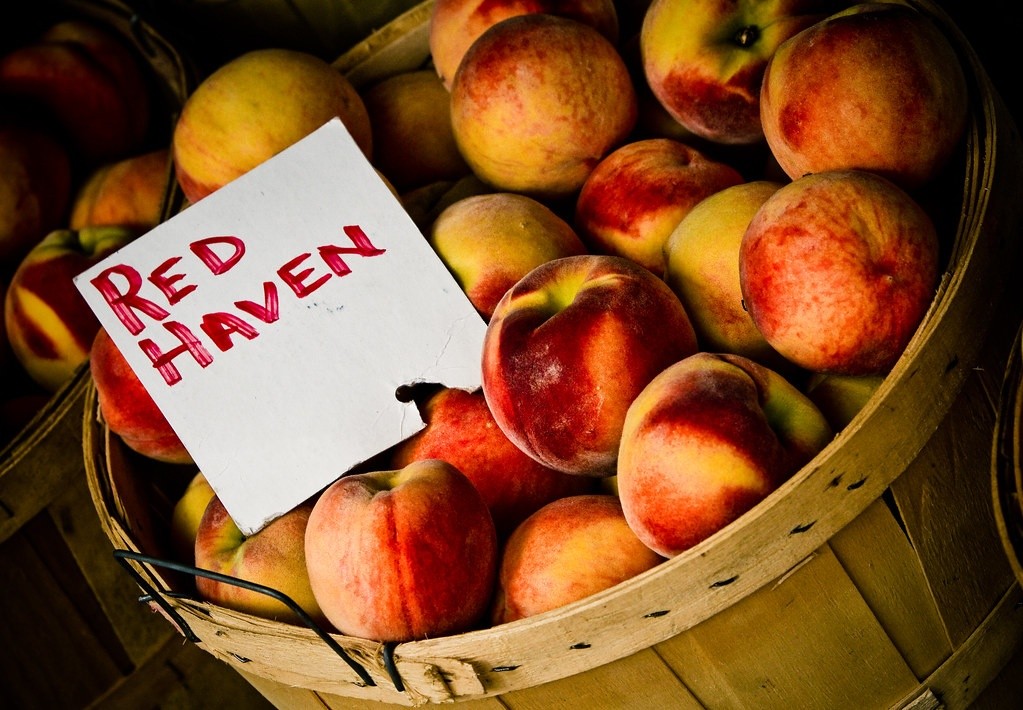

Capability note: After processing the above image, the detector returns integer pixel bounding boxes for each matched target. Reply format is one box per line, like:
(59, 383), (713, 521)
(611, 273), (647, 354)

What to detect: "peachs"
(0, 0), (950, 643)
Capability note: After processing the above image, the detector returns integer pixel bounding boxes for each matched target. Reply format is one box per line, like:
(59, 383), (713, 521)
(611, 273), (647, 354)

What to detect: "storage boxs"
(83, 4), (1023, 709)
